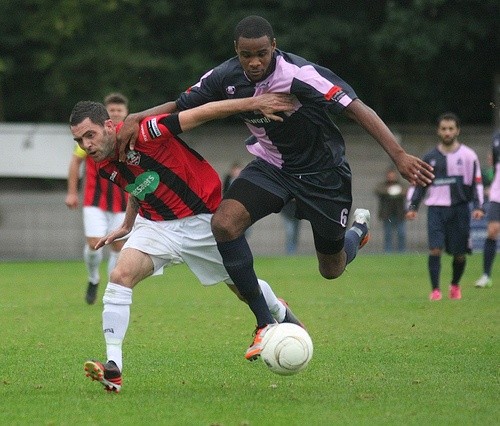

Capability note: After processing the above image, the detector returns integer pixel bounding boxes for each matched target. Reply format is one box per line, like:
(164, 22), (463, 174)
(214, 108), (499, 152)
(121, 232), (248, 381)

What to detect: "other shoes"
(86, 282), (99, 305)
(448, 283), (462, 299)
(474, 275), (491, 289)
(429, 288), (443, 301)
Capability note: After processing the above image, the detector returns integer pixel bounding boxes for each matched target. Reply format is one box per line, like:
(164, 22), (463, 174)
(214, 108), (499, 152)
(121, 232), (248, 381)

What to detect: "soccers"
(259, 323), (313, 377)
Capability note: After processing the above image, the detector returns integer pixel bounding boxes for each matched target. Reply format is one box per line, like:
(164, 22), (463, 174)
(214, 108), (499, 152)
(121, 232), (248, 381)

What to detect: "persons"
(478, 130), (500, 288)
(66, 93), (129, 304)
(69, 93), (302, 391)
(375, 168), (408, 251)
(283, 197), (301, 255)
(115, 16), (435, 363)
(408, 111), (486, 300)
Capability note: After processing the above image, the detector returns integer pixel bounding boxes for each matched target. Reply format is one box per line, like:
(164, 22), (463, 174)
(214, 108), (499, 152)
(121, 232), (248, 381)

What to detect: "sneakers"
(277, 297), (304, 328)
(84, 358), (122, 393)
(352, 208), (370, 249)
(245, 320), (280, 361)
